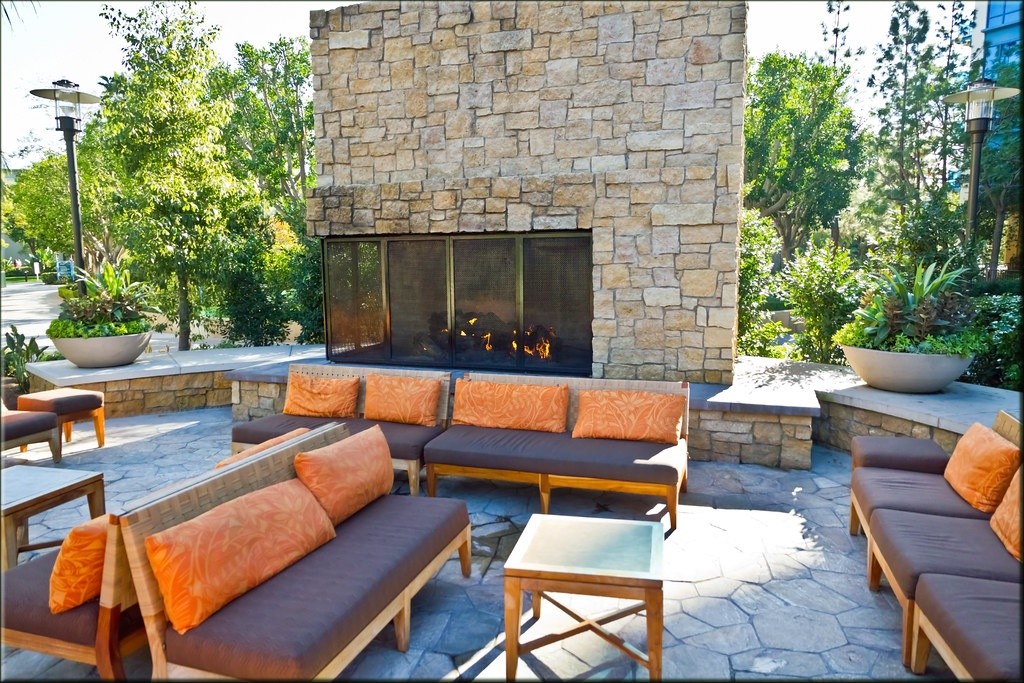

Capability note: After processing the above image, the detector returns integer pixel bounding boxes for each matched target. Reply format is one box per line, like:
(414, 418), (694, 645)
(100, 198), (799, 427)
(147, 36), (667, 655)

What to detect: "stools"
(18, 388), (105, 454)
(852, 433), (950, 536)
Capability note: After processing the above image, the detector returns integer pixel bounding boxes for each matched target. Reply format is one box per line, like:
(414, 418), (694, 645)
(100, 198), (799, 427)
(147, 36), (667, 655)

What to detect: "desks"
(503, 514), (665, 682)
(2, 464), (106, 576)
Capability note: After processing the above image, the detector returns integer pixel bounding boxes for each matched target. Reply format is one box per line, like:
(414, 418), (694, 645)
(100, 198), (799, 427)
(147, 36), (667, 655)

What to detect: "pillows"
(48, 514), (110, 613)
(448, 380), (572, 432)
(213, 425), (309, 468)
(148, 485), (336, 636)
(362, 375), (442, 427)
(991, 468), (1022, 562)
(295, 426), (399, 519)
(945, 420), (1019, 513)
(282, 373), (361, 419)
(570, 391), (689, 439)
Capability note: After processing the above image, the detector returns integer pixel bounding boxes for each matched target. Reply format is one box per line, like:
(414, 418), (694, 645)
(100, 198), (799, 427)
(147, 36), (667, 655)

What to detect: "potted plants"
(831, 255), (983, 394)
(49, 274), (157, 369)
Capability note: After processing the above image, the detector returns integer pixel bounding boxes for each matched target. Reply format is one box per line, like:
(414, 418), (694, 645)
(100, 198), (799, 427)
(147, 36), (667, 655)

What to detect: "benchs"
(121, 426), (474, 682)
(230, 360), (453, 495)
(851, 406), (1022, 681)
(0, 397), (61, 465)
(429, 374), (697, 531)
(1, 418), (340, 682)
